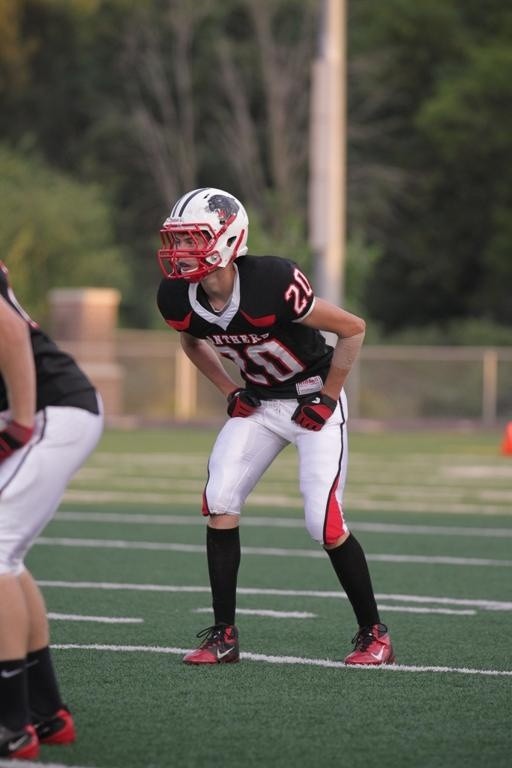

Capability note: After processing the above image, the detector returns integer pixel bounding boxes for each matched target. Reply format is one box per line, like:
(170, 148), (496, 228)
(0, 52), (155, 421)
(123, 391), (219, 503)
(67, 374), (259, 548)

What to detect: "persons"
(2, 264), (107, 761)
(154, 186), (398, 669)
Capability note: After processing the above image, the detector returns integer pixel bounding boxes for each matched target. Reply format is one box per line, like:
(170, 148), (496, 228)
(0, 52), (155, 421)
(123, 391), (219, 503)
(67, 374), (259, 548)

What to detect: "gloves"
(227, 386), (261, 417)
(291, 393), (336, 431)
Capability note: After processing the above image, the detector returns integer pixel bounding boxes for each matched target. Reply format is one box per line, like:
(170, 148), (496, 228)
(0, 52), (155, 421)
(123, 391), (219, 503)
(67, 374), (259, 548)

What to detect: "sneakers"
(182, 628), (238, 666)
(1, 721), (40, 761)
(34, 702), (77, 747)
(345, 624), (395, 664)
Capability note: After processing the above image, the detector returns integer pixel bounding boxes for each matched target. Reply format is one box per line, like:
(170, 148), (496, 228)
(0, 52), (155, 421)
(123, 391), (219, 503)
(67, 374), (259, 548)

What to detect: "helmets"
(157, 187), (249, 283)
